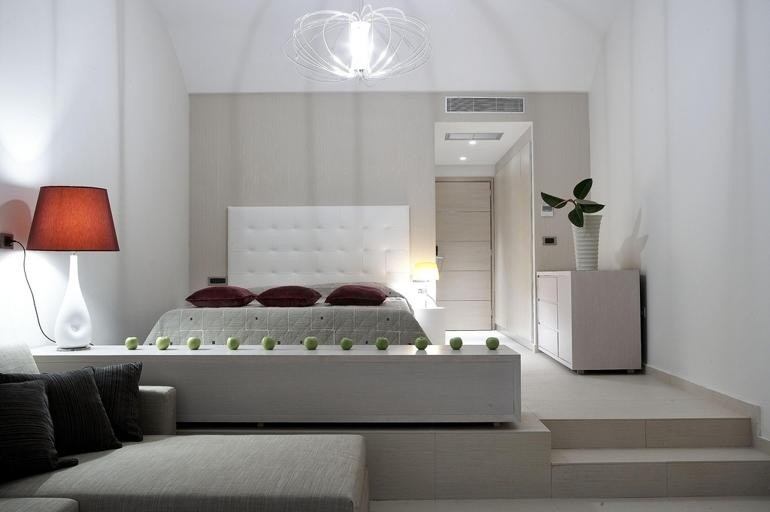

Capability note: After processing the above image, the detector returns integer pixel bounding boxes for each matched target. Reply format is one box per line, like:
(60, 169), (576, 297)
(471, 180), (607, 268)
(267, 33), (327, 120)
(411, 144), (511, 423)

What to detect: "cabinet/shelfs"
(534, 268), (642, 374)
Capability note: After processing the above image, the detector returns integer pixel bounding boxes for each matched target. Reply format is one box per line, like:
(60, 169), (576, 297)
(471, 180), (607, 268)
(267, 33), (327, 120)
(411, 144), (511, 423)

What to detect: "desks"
(412, 307), (446, 345)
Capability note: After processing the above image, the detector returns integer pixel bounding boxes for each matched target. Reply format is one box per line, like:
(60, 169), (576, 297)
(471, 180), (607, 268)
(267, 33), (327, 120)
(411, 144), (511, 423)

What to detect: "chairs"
(0, 343), (371, 510)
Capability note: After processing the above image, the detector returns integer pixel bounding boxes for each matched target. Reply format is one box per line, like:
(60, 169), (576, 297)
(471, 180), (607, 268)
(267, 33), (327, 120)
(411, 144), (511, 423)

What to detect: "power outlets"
(0, 232), (15, 249)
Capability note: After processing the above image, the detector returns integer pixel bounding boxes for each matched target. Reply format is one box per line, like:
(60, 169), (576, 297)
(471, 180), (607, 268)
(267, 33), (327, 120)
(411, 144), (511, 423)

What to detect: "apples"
(226, 337), (240, 350)
(155, 336), (170, 349)
(187, 336), (201, 350)
(304, 336), (318, 350)
(375, 337), (388, 350)
(340, 337), (353, 350)
(449, 336), (463, 350)
(415, 337), (428, 350)
(486, 337), (499, 350)
(262, 336), (275, 350)
(125, 336), (138, 349)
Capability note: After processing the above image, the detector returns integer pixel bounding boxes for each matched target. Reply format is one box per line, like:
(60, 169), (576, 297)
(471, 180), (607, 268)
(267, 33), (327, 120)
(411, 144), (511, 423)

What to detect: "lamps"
(277, 2), (433, 87)
(24, 184), (120, 351)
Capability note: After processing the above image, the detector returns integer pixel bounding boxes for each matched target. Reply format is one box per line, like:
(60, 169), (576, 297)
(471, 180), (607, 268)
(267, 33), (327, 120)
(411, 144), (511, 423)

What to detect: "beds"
(152, 205), (431, 344)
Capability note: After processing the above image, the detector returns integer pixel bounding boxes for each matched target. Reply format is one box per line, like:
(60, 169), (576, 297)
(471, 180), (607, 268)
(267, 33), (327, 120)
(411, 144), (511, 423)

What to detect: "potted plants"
(538, 176), (608, 271)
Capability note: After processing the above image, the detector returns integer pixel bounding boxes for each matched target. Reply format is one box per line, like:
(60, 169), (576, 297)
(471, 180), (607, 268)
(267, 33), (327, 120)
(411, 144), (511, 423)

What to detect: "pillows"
(0, 358), (145, 486)
(182, 286), (388, 308)
